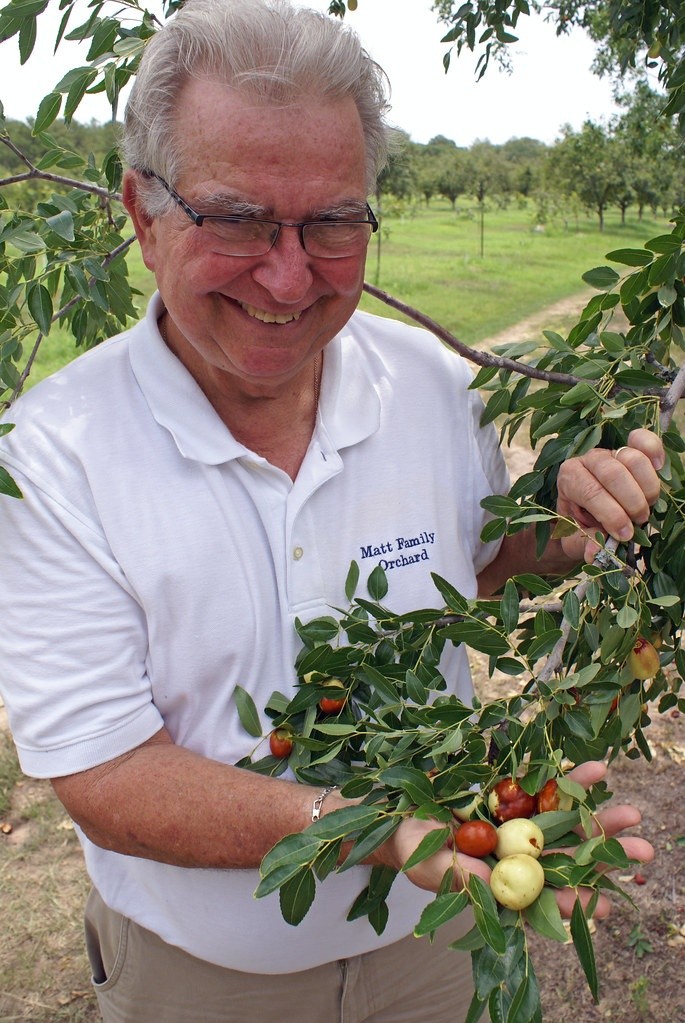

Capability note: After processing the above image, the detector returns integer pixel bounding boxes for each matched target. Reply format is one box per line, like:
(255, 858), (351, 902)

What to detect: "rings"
(615, 446), (627, 459)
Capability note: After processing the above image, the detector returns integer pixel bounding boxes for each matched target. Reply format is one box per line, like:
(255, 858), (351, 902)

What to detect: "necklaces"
(313, 356), (319, 422)
(311, 786), (340, 872)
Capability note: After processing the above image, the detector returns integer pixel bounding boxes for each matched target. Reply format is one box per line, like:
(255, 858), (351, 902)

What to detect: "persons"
(0, 0), (667, 1023)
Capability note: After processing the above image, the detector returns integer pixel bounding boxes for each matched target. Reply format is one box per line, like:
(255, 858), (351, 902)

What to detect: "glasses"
(141, 168), (379, 259)
